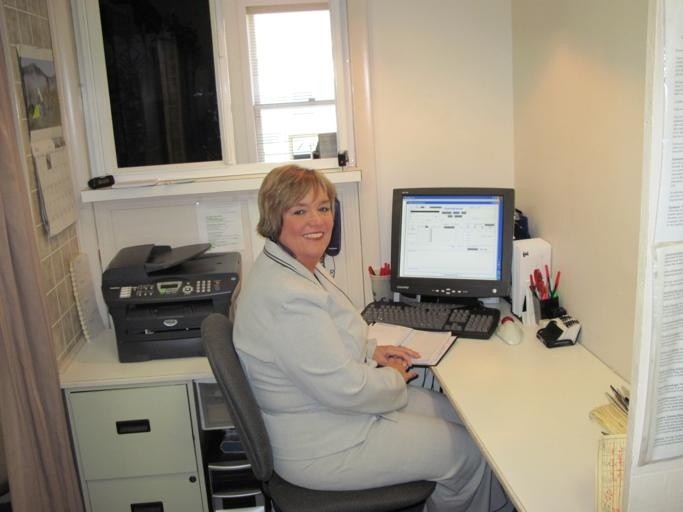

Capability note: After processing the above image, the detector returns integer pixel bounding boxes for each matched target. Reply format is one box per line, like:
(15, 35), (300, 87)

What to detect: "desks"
(60, 296), (631, 512)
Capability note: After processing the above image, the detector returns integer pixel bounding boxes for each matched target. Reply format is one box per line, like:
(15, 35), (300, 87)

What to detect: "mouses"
(498, 315), (525, 346)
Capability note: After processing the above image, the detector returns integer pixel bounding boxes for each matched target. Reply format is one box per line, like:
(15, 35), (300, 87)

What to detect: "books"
(366, 320), (459, 368)
(588, 402), (627, 435)
(594, 435), (626, 511)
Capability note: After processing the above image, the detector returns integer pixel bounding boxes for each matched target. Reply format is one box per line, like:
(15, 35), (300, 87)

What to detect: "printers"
(101, 241), (241, 363)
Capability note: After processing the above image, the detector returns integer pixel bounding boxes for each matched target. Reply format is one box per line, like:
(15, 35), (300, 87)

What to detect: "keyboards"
(361, 301), (502, 339)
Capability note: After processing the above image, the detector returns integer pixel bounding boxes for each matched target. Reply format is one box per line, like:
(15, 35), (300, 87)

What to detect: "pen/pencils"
(530, 264), (561, 300)
(368, 263), (391, 276)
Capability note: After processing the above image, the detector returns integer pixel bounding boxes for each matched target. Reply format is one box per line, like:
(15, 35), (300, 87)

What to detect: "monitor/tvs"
(388, 187), (514, 307)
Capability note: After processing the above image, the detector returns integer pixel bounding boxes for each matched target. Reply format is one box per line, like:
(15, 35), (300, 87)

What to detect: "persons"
(230, 166), (513, 511)
